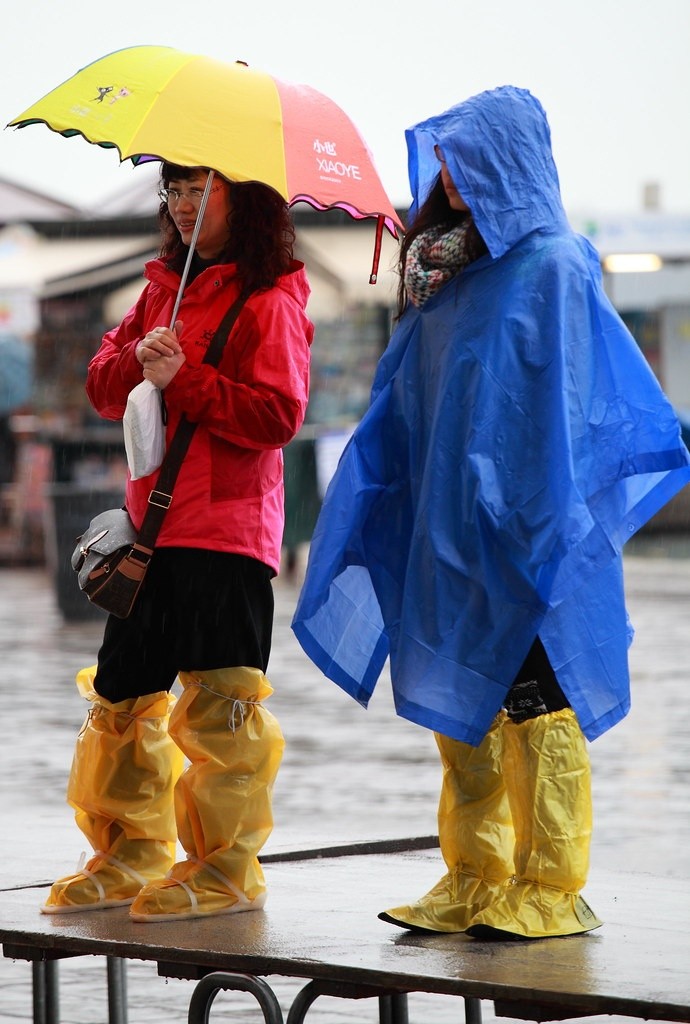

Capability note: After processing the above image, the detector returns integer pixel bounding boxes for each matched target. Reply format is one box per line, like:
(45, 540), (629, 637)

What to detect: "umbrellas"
(4, 44), (406, 331)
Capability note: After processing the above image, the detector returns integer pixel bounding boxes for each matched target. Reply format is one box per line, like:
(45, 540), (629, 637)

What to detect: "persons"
(42, 159), (314, 923)
(290, 85), (690, 939)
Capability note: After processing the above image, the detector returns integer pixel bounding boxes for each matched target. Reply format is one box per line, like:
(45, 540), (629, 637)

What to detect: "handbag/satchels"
(122, 379), (164, 481)
(70, 508), (153, 620)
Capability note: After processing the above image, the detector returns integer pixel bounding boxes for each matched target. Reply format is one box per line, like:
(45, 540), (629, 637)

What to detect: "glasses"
(156, 180), (228, 206)
(433, 144), (447, 162)
